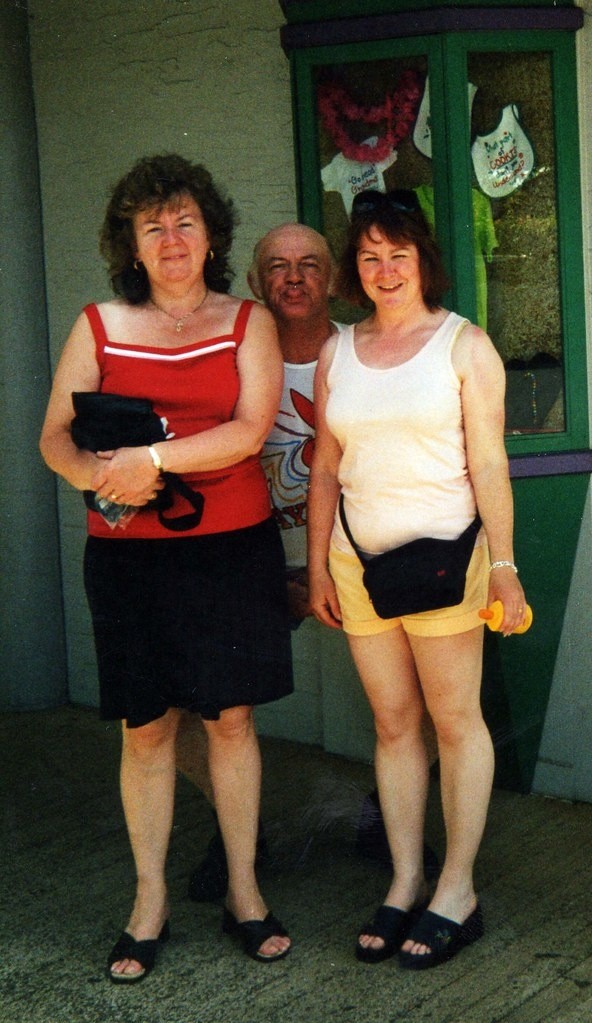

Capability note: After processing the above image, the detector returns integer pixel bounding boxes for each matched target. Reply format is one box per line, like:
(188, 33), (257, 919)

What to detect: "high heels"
(354, 894), (432, 965)
(398, 901), (483, 971)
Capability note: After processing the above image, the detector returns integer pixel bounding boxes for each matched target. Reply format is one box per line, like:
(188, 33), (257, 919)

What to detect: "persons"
(37, 154), (291, 983)
(302, 189), (526, 965)
(175, 223), (440, 902)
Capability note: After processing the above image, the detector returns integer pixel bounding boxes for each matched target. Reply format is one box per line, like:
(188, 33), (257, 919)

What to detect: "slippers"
(106, 915), (169, 983)
(220, 906), (291, 962)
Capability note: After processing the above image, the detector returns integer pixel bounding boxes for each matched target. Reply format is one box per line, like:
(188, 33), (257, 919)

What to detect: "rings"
(518, 608), (523, 613)
(111, 495), (118, 499)
(151, 494), (155, 500)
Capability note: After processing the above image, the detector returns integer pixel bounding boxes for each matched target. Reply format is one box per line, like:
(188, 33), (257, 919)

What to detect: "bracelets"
(149, 446), (164, 473)
(490, 561), (519, 574)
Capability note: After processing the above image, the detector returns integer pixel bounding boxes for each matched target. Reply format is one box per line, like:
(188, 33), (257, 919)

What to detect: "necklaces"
(151, 289), (210, 332)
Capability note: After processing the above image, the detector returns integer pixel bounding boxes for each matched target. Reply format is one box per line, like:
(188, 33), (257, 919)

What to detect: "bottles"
(478, 599), (532, 634)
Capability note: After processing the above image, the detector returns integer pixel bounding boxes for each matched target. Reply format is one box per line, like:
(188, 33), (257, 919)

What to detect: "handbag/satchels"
(69, 390), (204, 531)
(362, 530), (477, 620)
